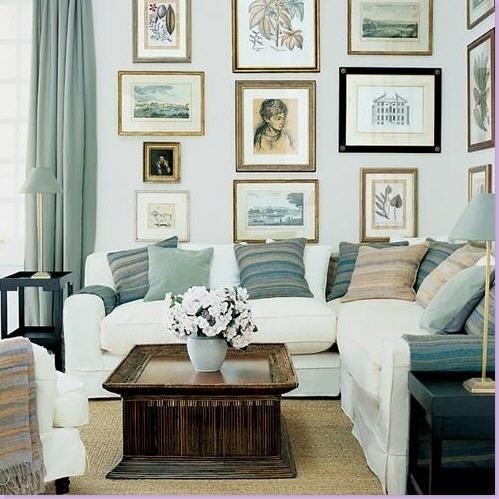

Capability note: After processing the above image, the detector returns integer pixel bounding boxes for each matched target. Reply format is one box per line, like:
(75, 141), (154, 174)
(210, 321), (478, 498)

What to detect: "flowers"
(165, 285), (258, 352)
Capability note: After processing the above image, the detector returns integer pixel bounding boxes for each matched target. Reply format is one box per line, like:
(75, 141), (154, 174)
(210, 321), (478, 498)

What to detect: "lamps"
(449, 193), (497, 394)
(19, 166), (63, 278)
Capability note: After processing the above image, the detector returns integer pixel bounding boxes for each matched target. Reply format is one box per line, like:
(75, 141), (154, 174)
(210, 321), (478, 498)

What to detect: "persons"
(151, 150), (171, 175)
(256, 98), (298, 153)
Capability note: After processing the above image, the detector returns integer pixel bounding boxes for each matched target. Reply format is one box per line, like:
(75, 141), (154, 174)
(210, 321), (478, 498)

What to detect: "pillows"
(233, 238), (314, 300)
(341, 242), (430, 302)
(107, 235), (178, 304)
(326, 240), (409, 302)
(144, 244), (214, 302)
(416, 236), (495, 336)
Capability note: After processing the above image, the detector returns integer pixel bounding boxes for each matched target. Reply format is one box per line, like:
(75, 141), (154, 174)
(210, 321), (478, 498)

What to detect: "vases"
(185, 337), (227, 372)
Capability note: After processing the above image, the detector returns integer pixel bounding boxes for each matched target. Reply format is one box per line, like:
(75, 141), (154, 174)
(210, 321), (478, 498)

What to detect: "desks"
(0, 272), (74, 374)
(406, 369), (494, 494)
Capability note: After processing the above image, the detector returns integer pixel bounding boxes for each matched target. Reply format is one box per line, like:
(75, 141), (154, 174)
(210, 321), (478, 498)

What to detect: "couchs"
(64, 234), (497, 495)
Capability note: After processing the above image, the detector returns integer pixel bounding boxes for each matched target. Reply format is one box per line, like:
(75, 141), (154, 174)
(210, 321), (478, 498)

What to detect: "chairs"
(0, 336), (89, 496)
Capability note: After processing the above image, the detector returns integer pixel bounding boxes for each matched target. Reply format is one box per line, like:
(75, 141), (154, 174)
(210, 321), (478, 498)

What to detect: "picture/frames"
(132, 1), (191, 63)
(468, 32), (497, 151)
(131, 187), (191, 243)
(347, 2), (433, 55)
(229, 1), (319, 74)
(141, 140), (182, 185)
(230, 178), (321, 244)
(337, 67), (443, 154)
(468, 166), (491, 205)
(465, 0), (494, 28)
(233, 79), (316, 174)
(359, 170), (419, 245)
(117, 70), (205, 138)
